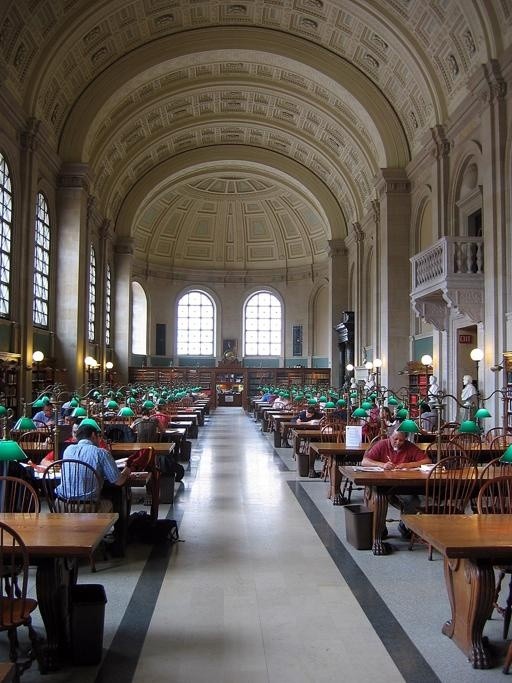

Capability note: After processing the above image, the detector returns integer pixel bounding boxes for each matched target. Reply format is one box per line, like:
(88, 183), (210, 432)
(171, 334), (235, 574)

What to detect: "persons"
(53, 419), (132, 544)
(46, 408), (80, 444)
(33, 403), (62, 428)
(256, 376), (486, 536)
(130, 408), (165, 443)
(151, 407), (170, 429)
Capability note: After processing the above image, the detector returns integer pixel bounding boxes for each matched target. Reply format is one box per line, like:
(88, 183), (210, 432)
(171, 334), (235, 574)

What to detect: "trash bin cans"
(158, 471), (176, 504)
(247, 403), (269, 432)
(274, 431), (281, 448)
(180, 441), (191, 462)
(343, 505), (374, 550)
(69, 584), (108, 667)
(296, 453), (309, 477)
(190, 425), (198, 439)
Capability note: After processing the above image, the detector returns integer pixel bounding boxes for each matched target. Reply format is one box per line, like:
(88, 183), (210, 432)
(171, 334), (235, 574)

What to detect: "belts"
(53, 493), (99, 507)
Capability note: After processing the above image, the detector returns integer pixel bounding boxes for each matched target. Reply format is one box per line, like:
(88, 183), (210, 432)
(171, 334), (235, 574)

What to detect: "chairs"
(1, 379), (214, 649)
(246, 380), (511, 661)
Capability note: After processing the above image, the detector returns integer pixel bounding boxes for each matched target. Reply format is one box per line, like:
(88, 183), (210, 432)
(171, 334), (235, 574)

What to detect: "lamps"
(344, 362), (356, 373)
(420, 353), (433, 390)
(30, 350), (46, 366)
(468, 349), (486, 398)
(365, 358), (384, 396)
(83, 356), (115, 378)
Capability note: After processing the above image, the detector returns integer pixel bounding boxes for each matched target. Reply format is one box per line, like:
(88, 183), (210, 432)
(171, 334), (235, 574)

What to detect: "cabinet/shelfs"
(125, 364), (333, 388)
